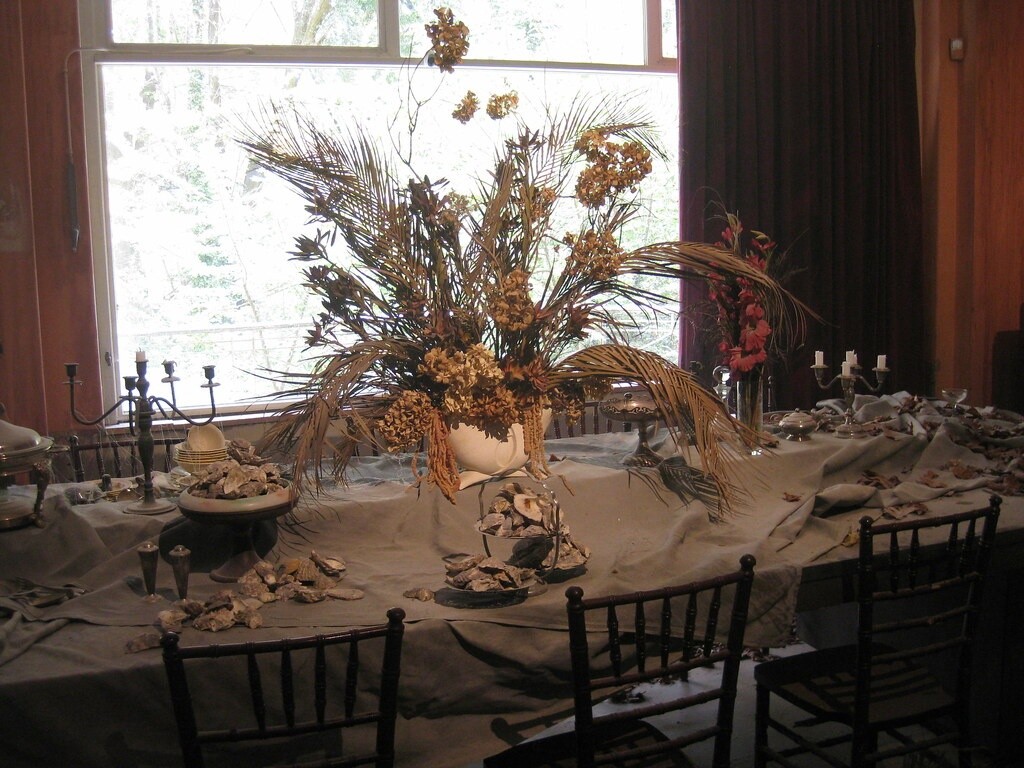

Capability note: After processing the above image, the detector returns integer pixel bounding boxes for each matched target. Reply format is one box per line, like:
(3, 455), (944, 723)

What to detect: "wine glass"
(942, 387), (967, 419)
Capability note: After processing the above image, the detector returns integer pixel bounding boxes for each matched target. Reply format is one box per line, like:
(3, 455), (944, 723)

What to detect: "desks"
(0, 405), (1024, 768)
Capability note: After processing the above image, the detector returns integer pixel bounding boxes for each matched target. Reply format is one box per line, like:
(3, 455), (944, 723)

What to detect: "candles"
(845, 350), (855, 365)
(813, 348), (825, 364)
(876, 353), (887, 369)
(841, 360), (851, 376)
(135, 343), (147, 363)
(855, 352), (859, 365)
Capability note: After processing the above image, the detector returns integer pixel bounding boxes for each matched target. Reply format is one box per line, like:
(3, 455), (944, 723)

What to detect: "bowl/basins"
(172, 424), (234, 473)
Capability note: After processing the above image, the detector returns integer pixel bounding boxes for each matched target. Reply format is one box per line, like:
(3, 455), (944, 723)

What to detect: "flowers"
(237, 9), (810, 531)
(695, 185), (807, 380)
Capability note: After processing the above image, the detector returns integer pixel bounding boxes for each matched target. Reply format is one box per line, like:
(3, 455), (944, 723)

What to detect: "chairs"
(155, 602), (409, 768)
(552, 386), (631, 440)
(750, 490), (1005, 768)
(341, 409), (425, 457)
(65, 430), (221, 485)
(482, 550), (759, 768)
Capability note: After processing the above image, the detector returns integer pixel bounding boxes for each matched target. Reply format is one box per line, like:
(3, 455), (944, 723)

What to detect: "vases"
(734, 364), (771, 453)
(445, 401), (554, 477)
